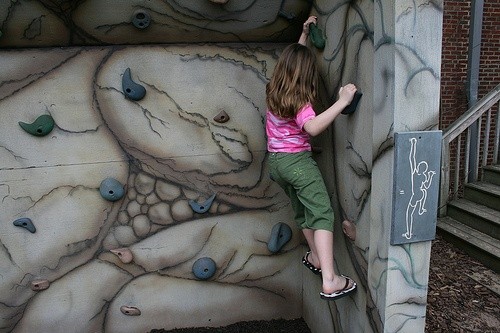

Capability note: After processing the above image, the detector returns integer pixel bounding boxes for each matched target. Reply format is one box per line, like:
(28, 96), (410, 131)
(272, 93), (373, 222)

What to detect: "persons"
(264, 15), (359, 301)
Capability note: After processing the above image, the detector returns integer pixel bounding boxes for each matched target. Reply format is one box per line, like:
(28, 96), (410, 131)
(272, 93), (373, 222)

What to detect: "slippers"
(301, 250), (323, 275)
(320, 275), (357, 301)
